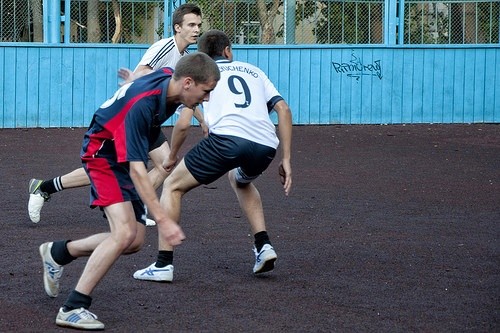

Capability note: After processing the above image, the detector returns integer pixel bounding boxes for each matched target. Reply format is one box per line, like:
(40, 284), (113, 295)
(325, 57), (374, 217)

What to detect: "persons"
(25, 53), (222, 330)
(133, 30), (295, 281)
(26, 4), (202, 227)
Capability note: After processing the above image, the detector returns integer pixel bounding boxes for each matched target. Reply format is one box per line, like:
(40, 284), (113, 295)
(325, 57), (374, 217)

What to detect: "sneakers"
(28, 178), (48, 223)
(252, 243), (277, 274)
(56, 307), (104, 329)
(133, 262), (174, 281)
(39, 242), (63, 297)
(146, 219), (156, 226)
